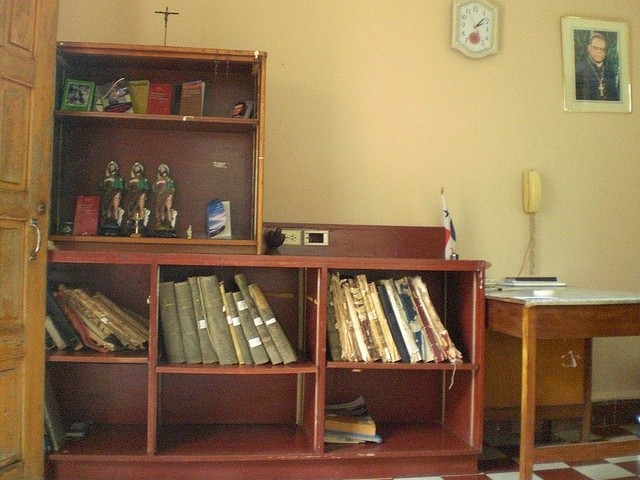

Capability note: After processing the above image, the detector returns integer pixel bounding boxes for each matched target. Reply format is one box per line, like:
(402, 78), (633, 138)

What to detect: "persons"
(100, 160), (124, 223)
(153, 163), (175, 226)
(574, 32), (622, 102)
(125, 162), (150, 225)
(230, 102), (247, 118)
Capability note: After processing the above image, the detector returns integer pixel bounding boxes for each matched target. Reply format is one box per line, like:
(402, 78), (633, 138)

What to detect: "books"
(46, 285), (150, 356)
(498, 276), (567, 292)
(158, 274), (297, 365)
(45, 369), (90, 453)
(327, 270), (463, 364)
(324, 394), (382, 444)
(73, 195), (100, 237)
(179, 81), (204, 116)
(127, 80), (149, 113)
(94, 79), (132, 113)
(207, 200), (232, 239)
(146, 82), (174, 115)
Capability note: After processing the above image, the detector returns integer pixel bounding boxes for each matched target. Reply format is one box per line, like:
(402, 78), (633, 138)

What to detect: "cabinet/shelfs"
(46, 251), (486, 480)
(49, 41), (267, 255)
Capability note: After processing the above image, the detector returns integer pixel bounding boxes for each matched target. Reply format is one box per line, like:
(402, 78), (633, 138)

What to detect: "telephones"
(523, 169), (540, 277)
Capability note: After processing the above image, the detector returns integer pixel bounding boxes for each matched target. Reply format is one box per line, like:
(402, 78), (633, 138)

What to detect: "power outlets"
(265, 228), (301, 246)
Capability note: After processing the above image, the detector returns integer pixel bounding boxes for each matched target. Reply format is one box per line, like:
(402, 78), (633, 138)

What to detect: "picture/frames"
(561, 16), (632, 113)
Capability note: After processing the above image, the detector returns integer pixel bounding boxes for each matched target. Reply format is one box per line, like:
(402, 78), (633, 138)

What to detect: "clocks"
(451, 0), (500, 59)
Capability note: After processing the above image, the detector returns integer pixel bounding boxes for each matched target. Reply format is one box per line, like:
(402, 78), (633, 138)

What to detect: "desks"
(487, 294), (640, 480)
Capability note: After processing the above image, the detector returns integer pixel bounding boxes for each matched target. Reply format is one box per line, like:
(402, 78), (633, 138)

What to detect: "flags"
(442, 186), (456, 260)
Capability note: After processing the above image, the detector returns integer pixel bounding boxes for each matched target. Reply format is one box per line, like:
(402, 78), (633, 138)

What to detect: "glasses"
(592, 45), (606, 52)
